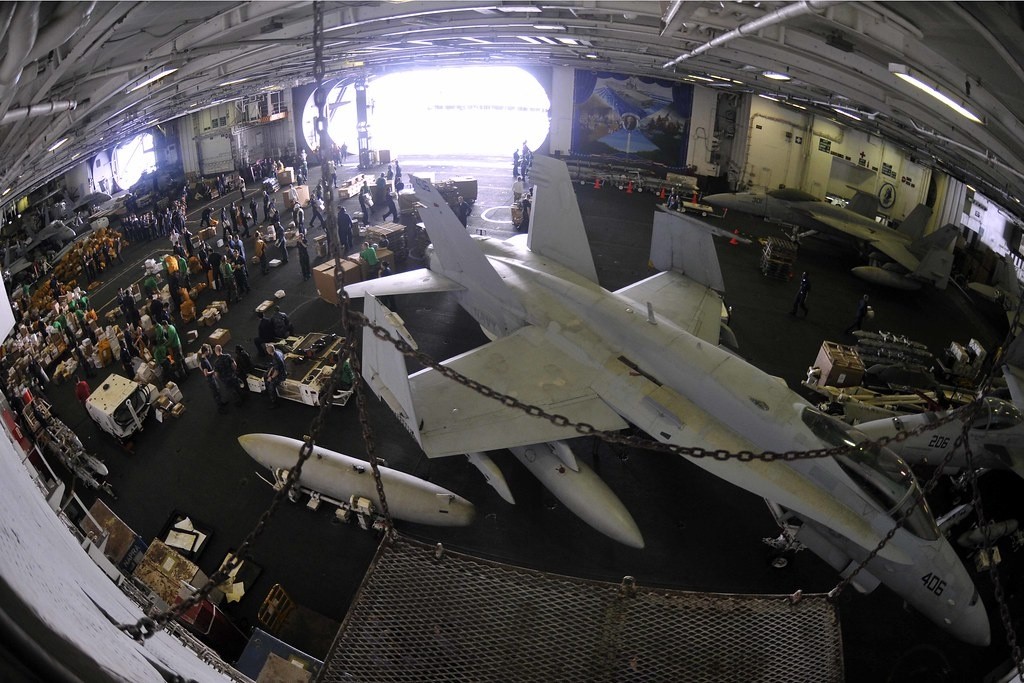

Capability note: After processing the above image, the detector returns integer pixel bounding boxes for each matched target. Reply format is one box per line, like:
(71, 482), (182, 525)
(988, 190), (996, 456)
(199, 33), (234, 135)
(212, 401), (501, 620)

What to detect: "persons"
(0, 138), (534, 420)
(789, 272), (811, 316)
(667, 187), (684, 213)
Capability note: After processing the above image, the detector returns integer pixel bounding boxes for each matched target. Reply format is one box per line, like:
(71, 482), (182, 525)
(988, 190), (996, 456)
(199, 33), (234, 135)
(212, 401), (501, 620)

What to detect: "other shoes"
(383, 215), (385, 221)
(310, 224), (314, 227)
(305, 278), (307, 281)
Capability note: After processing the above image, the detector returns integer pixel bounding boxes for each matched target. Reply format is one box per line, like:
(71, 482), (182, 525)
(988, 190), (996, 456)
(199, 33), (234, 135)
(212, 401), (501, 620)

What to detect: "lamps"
(260, 19), (283, 34)
(886, 62), (986, 124)
(124, 63), (179, 96)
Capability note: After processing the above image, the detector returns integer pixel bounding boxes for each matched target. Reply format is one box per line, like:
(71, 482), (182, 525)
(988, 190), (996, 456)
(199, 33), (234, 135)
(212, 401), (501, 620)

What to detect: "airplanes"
(1, 201), (79, 284)
(235, 154), (1024, 648)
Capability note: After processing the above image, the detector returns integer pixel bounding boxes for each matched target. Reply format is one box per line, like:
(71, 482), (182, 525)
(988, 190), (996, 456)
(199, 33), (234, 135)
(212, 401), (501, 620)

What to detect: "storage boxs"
(208, 328), (232, 347)
(0, 284), (140, 379)
(137, 363), (152, 381)
(282, 185), (311, 210)
(278, 167), (295, 185)
(197, 316), (206, 326)
(312, 258), (361, 307)
(313, 235), (330, 257)
(399, 177), (477, 211)
(365, 221), (408, 261)
(138, 381), (185, 423)
(346, 248), (397, 280)
(255, 300), (274, 312)
(337, 173), (377, 199)
(813, 344), (866, 388)
(207, 304), (224, 317)
(202, 309), (217, 327)
(378, 150), (390, 163)
(198, 227), (218, 241)
(212, 301), (229, 314)
